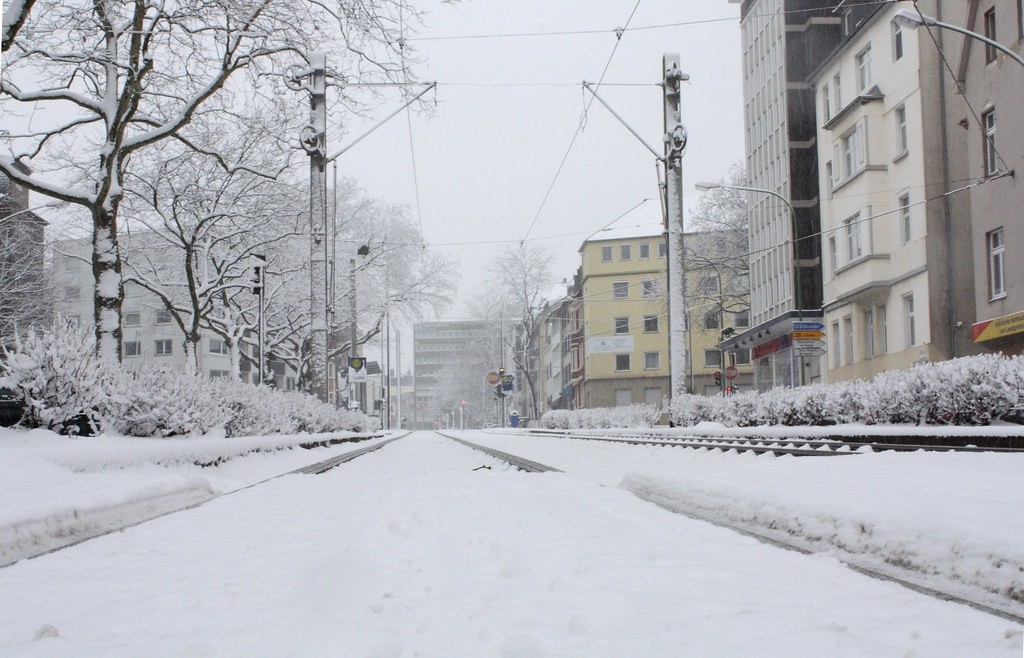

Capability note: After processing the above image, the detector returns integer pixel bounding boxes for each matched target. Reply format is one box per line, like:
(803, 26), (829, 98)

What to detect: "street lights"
(693, 181), (806, 387)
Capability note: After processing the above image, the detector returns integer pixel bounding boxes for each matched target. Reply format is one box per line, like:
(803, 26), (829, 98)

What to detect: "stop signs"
(487, 372), (500, 385)
(725, 366), (738, 381)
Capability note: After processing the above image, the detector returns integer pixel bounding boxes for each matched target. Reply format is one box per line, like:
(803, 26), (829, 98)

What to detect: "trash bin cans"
(510, 410), (519, 428)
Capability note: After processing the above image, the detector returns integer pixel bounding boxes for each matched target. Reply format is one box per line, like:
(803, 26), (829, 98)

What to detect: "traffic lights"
(495, 384), (502, 398)
(713, 369), (721, 387)
